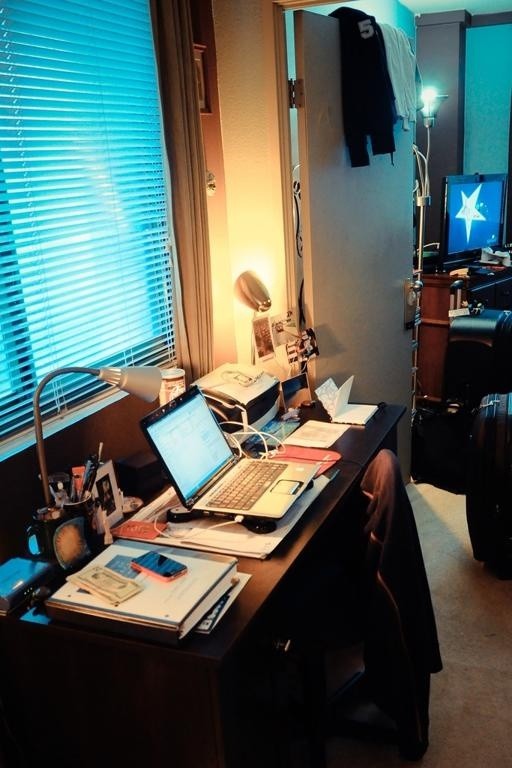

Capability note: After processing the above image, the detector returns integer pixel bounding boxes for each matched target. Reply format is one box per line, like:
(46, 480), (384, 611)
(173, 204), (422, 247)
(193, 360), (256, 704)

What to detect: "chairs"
(306, 448), (430, 761)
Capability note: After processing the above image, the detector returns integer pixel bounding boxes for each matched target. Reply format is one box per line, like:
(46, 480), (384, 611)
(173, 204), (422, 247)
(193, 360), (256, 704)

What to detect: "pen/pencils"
(39, 443), (103, 503)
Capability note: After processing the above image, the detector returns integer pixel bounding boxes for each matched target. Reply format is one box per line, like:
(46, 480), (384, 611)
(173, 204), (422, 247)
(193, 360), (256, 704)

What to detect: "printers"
(189, 363), (300, 449)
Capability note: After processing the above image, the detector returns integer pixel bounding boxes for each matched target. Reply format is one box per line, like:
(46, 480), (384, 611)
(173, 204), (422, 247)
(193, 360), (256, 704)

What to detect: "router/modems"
(0, 557), (53, 611)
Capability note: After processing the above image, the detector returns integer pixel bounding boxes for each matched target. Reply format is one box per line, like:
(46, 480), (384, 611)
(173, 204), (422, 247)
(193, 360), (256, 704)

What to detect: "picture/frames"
(191, 44), (214, 114)
(92, 460), (123, 534)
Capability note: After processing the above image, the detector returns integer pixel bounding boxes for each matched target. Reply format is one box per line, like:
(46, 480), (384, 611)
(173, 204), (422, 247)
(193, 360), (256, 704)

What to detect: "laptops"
(139, 385), (322, 522)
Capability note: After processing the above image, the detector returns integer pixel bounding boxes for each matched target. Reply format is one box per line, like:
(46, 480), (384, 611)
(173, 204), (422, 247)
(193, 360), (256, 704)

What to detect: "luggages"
(466, 394), (512, 579)
(443, 280), (512, 401)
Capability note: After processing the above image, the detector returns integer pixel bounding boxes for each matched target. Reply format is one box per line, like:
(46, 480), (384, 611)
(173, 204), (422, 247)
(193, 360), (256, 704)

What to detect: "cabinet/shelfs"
(413, 272), (512, 402)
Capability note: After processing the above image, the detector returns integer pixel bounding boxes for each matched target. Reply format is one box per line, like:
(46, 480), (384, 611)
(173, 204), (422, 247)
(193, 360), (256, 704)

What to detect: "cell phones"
(130, 550), (188, 583)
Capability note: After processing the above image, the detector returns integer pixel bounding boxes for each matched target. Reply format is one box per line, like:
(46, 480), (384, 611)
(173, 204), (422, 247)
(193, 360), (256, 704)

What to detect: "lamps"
(234, 270), (272, 366)
(413, 86), (441, 429)
(34, 367), (162, 506)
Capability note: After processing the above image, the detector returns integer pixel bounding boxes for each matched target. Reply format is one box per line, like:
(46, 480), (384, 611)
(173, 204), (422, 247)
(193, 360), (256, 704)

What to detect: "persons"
(93, 474), (118, 520)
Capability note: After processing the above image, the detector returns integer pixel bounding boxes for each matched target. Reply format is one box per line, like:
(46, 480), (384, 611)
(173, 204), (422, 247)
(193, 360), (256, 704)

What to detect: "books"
(245, 420), (300, 447)
(44, 539), (251, 648)
(314, 375), (379, 425)
(1, 557), (55, 617)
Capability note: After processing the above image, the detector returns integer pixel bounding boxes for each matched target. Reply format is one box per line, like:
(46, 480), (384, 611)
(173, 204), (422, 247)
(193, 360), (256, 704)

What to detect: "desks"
(0, 401), (408, 768)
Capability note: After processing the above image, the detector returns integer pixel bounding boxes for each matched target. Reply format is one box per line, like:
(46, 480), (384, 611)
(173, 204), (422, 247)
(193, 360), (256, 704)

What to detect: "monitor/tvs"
(439, 172), (509, 270)
